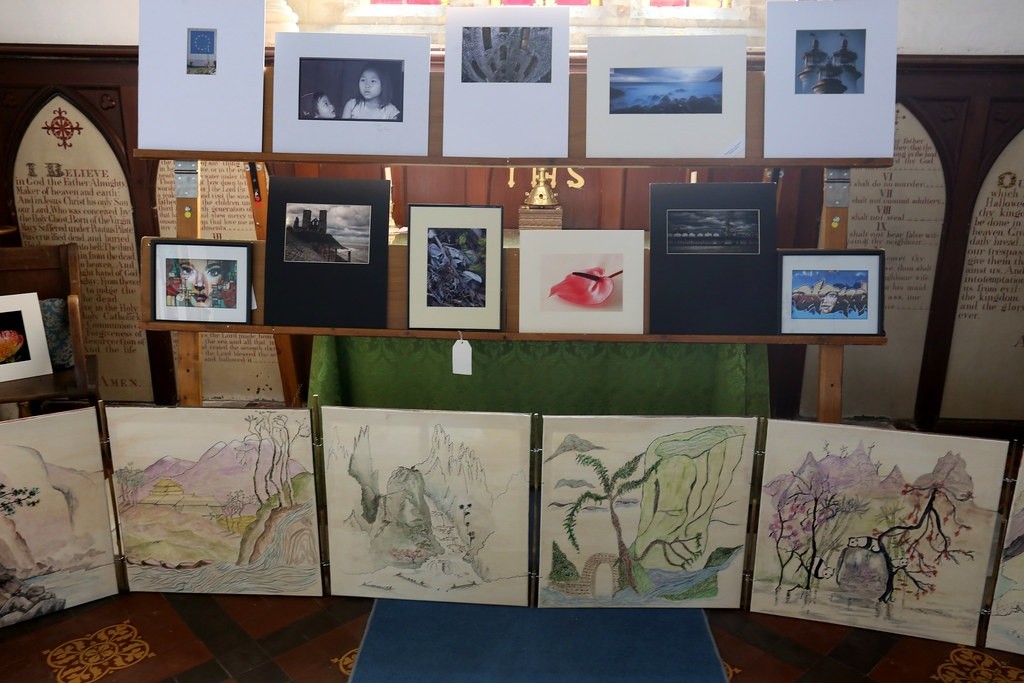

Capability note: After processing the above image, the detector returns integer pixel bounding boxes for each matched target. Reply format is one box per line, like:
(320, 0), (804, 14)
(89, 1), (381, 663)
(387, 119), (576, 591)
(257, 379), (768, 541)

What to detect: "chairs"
(0, 242), (110, 451)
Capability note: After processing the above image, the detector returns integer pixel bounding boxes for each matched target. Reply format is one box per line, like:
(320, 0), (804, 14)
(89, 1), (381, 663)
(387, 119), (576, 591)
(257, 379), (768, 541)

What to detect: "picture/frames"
(150, 239), (252, 325)
(406, 202), (504, 331)
(0, 291), (54, 392)
(778, 249), (888, 334)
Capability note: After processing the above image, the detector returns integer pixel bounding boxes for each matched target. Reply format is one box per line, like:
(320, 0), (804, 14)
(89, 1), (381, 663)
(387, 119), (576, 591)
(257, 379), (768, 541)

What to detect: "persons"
(300, 63), (401, 119)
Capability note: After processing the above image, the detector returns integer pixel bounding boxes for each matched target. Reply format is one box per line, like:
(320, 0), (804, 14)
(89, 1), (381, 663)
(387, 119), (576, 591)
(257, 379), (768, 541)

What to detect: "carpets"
(348, 601), (731, 683)
